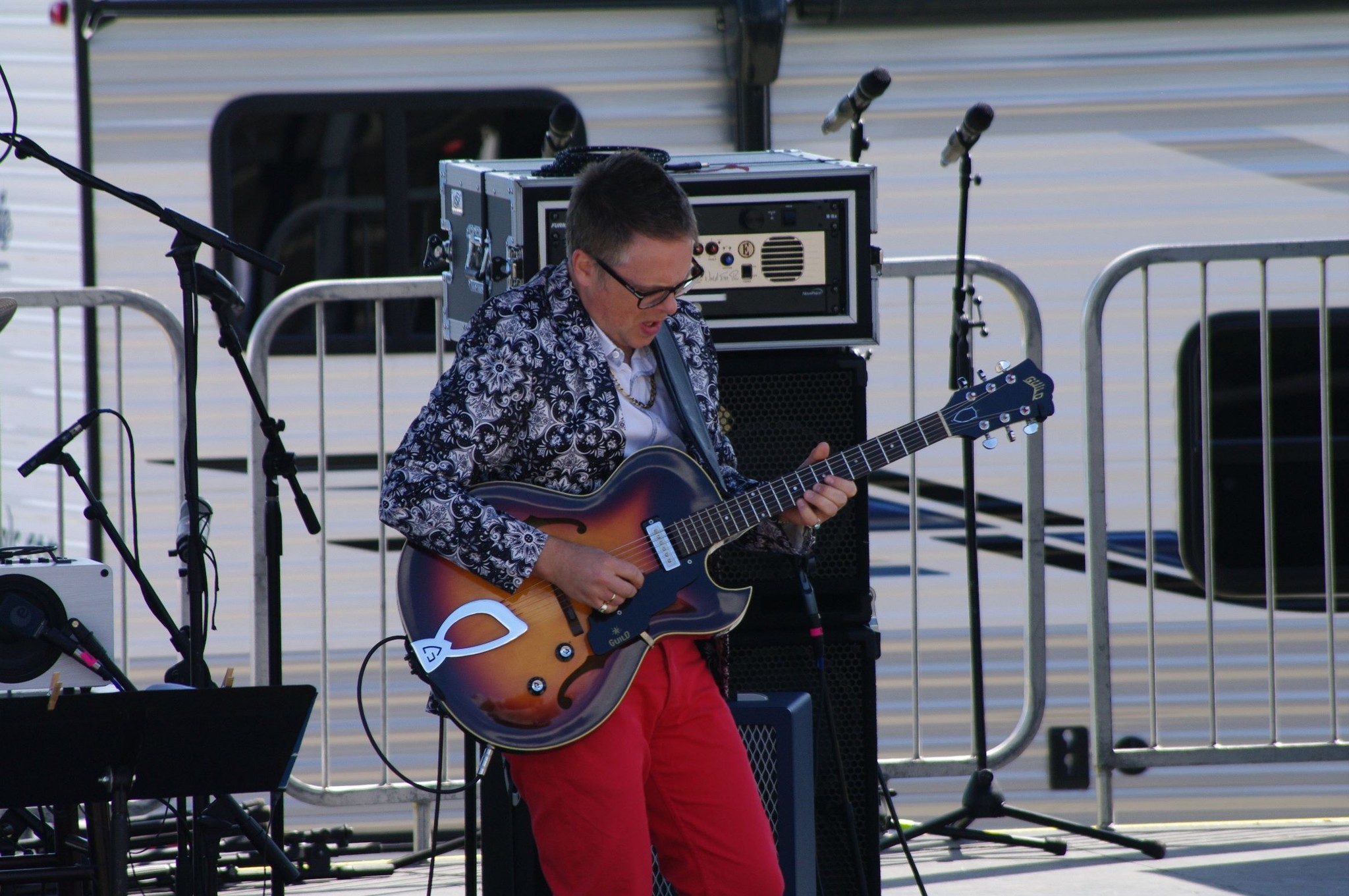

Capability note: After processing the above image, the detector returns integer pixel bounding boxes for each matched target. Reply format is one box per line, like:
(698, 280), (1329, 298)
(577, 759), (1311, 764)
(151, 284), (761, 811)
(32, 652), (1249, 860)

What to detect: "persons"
(378, 154), (858, 896)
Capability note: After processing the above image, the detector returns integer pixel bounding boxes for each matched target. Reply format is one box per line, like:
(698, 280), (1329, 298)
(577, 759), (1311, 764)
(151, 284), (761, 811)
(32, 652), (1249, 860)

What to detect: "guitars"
(396, 356), (1058, 753)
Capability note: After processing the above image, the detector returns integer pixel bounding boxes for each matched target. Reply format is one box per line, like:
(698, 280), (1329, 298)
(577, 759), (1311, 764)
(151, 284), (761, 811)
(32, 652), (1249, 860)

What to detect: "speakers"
(481, 347), (881, 896)
(0, 556), (115, 692)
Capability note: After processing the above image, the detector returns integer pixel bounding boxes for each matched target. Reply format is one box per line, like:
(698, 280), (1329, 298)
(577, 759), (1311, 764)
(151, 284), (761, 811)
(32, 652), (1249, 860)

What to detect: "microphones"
(821, 68), (892, 135)
(0, 591), (115, 681)
(940, 103), (993, 169)
(17, 408), (99, 478)
(541, 104), (580, 159)
(175, 497), (213, 564)
(795, 568), (827, 671)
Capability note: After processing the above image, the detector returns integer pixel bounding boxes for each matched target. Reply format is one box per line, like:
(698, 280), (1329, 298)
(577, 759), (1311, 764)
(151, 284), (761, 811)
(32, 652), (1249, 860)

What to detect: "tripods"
(881, 130), (1165, 858)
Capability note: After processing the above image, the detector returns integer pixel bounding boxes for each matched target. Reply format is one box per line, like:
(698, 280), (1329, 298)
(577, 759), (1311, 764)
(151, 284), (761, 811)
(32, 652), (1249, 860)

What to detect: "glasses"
(581, 247), (706, 310)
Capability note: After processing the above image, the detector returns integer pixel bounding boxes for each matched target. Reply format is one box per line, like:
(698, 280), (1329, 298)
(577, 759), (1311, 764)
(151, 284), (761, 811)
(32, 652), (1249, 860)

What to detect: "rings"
(607, 592), (616, 605)
(806, 517), (821, 529)
(598, 601), (607, 614)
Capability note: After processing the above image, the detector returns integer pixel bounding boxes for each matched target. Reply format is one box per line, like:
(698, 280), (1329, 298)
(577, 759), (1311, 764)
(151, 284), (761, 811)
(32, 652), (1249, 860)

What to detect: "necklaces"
(610, 370), (657, 410)
